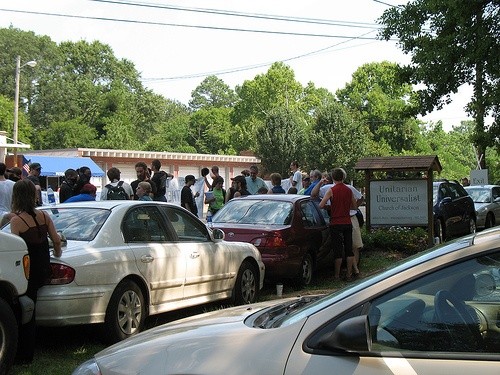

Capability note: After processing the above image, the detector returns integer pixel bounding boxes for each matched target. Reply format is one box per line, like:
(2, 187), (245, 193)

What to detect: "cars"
(462, 185), (500, 228)
(1, 228), (35, 375)
(4, 201), (265, 342)
(432, 180), (477, 242)
(205, 194), (362, 289)
(70, 224), (500, 375)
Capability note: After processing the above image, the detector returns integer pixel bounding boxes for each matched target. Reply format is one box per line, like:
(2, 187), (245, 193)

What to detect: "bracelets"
(55, 250), (62, 253)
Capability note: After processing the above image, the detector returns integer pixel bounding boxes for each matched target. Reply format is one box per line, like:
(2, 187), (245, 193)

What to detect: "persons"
(0, 162), (17, 224)
(0, 213), (18, 229)
(59, 169), (79, 203)
(267, 160), (332, 198)
(75, 166), (91, 192)
(181, 175), (200, 219)
(6, 167), (22, 182)
(203, 165), (269, 221)
(150, 159), (174, 202)
(62, 183), (97, 203)
(461, 177), (470, 187)
(318, 168), (357, 282)
(10, 178), (62, 367)
(100, 167), (134, 200)
(130, 162), (158, 201)
(26, 163), (43, 206)
(311, 172), (364, 279)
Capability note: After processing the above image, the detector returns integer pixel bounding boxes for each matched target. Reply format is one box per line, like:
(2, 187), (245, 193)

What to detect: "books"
(205, 192), (214, 200)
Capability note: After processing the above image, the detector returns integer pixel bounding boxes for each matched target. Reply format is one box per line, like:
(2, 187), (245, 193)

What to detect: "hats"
(6, 167), (21, 175)
(201, 168), (209, 177)
(185, 175), (198, 181)
(29, 163), (42, 170)
(83, 184), (97, 191)
(230, 176), (245, 180)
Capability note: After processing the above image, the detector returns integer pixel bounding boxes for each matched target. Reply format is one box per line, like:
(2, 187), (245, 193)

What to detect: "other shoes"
(342, 275), (352, 282)
(354, 272), (365, 279)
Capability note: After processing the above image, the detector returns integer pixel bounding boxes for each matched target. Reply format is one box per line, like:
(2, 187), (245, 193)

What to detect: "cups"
(277, 285), (283, 296)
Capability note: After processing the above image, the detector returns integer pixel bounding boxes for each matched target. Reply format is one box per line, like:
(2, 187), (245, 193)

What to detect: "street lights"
(11, 56), (37, 170)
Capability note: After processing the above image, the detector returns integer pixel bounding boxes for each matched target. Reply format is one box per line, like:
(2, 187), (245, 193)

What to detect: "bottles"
(47, 186), (56, 206)
(207, 209), (212, 227)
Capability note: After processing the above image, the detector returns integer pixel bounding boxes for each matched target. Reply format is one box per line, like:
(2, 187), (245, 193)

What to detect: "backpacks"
(105, 181), (129, 200)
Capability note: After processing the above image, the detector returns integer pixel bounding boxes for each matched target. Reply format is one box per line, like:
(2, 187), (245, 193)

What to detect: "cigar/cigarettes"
(198, 192), (200, 193)
